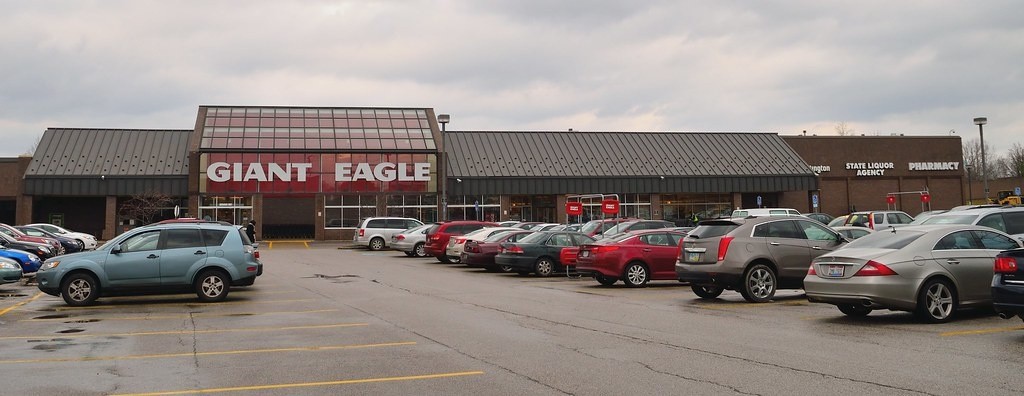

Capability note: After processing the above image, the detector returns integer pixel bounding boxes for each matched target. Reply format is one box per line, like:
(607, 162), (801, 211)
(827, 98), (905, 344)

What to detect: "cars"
(828, 215), (867, 229)
(460, 231), (535, 273)
(12, 226), (84, 252)
(25, 224), (98, 250)
(990, 248), (1024, 320)
(495, 217), (698, 238)
(803, 213), (836, 224)
(494, 231), (599, 278)
(0, 223), (62, 256)
(0, 244), (42, 272)
(917, 190), (1024, 242)
(423, 221), (500, 263)
(0, 231), (56, 261)
(446, 226), (525, 264)
(802, 225), (1024, 323)
(576, 229), (690, 288)
(389, 223), (439, 257)
(0, 256), (25, 283)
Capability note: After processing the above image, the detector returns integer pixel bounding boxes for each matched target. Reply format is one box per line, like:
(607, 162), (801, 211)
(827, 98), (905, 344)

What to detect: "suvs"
(845, 211), (914, 231)
(353, 217), (426, 251)
(34, 221), (259, 304)
(676, 215), (853, 302)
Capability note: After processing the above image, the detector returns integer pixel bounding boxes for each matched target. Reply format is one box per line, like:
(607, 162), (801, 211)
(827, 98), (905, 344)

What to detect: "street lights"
(437, 113), (450, 218)
(974, 116), (995, 205)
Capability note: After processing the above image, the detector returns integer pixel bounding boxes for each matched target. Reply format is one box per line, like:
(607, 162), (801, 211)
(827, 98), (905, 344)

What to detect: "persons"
(650, 235), (659, 242)
(246, 220), (256, 243)
(524, 225), (529, 230)
(689, 209), (699, 227)
(226, 209), (234, 224)
(553, 235), (563, 245)
(942, 234), (956, 249)
(203, 212), (211, 222)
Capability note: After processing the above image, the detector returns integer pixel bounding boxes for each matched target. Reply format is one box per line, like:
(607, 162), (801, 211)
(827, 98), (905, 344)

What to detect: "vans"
(732, 207), (803, 220)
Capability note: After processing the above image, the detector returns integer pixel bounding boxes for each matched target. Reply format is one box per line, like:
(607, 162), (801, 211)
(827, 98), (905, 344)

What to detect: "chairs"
(941, 235), (957, 248)
(650, 235), (659, 244)
